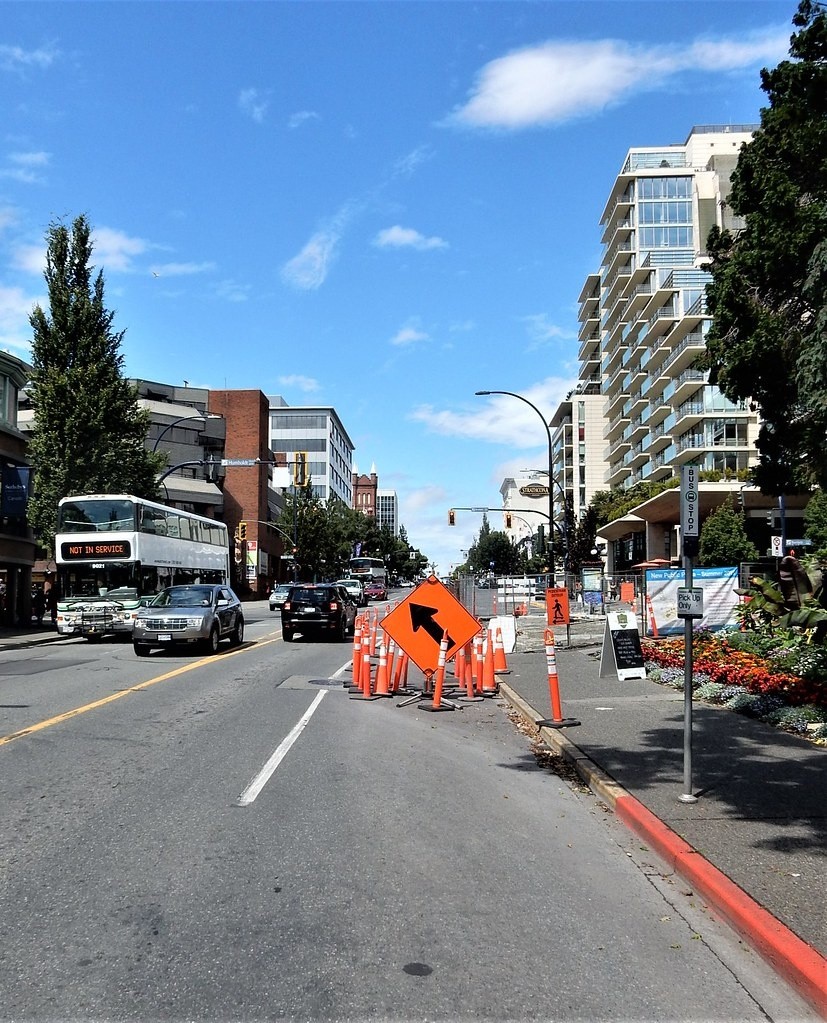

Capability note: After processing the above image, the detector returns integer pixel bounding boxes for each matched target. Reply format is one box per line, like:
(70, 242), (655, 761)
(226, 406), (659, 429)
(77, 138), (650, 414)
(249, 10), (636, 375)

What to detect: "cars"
(364, 584), (388, 601)
(268, 583), (295, 611)
(336, 580), (366, 607)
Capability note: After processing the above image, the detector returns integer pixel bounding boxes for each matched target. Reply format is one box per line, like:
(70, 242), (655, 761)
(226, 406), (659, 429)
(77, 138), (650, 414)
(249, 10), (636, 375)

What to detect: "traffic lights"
(448, 510), (455, 526)
(239, 523), (247, 540)
(736, 491), (746, 507)
(505, 513), (513, 529)
(767, 510), (775, 528)
(785, 548), (800, 560)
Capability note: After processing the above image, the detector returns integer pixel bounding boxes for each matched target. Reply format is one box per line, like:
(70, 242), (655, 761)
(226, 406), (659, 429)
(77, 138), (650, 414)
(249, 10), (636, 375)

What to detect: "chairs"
(310, 595), (319, 601)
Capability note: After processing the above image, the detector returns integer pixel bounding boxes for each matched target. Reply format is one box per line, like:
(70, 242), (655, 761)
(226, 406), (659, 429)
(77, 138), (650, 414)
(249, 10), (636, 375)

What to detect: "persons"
(33, 584), (59, 625)
(88, 579), (108, 596)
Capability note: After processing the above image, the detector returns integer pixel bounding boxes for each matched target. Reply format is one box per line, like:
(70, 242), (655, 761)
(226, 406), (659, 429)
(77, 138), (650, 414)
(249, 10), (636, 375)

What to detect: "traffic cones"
(471, 642), (479, 678)
(492, 628), (512, 674)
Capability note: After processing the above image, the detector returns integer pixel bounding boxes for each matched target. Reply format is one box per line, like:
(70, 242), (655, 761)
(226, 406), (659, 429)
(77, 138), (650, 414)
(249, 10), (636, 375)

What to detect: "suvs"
(132, 584), (245, 657)
(281, 581), (357, 642)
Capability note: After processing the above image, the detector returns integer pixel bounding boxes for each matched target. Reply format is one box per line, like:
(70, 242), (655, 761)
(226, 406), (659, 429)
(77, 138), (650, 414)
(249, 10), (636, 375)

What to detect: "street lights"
(151, 414), (222, 456)
(475, 390), (555, 589)
(519, 468), (570, 551)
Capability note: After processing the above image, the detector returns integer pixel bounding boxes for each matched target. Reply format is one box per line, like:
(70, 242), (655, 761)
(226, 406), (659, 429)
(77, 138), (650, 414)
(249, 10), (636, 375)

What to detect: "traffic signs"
(546, 587), (570, 626)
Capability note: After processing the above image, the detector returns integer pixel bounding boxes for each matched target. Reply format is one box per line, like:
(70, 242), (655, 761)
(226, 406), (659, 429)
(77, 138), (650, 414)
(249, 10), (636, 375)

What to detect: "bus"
(349, 557), (386, 592)
(54, 494), (231, 643)
